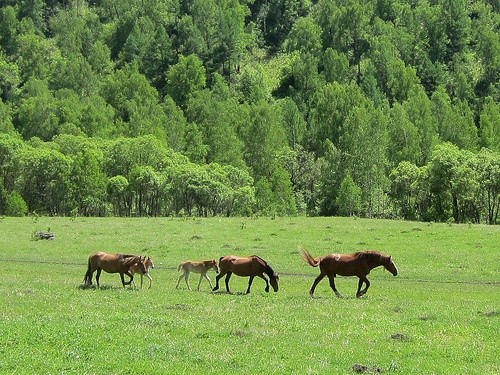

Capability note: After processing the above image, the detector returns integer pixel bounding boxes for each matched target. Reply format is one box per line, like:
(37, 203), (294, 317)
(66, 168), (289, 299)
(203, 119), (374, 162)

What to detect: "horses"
(175, 259), (220, 293)
(82, 252), (148, 291)
(297, 243), (398, 299)
(211, 255), (280, 295)
(117, 253), (154, 291)
